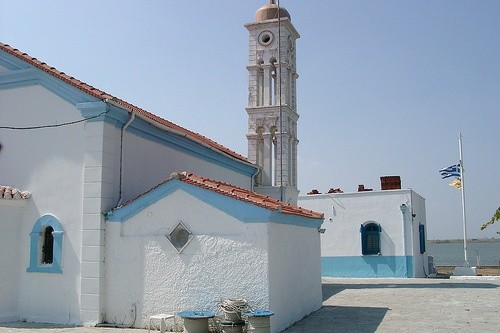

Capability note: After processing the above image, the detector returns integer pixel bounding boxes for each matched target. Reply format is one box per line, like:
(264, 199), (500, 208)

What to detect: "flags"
(448, 177), (462, 190)
(439, 163), (461, 179)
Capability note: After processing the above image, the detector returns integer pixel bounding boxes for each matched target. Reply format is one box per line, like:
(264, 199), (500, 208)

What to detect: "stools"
(148, 313), (177, 333)
